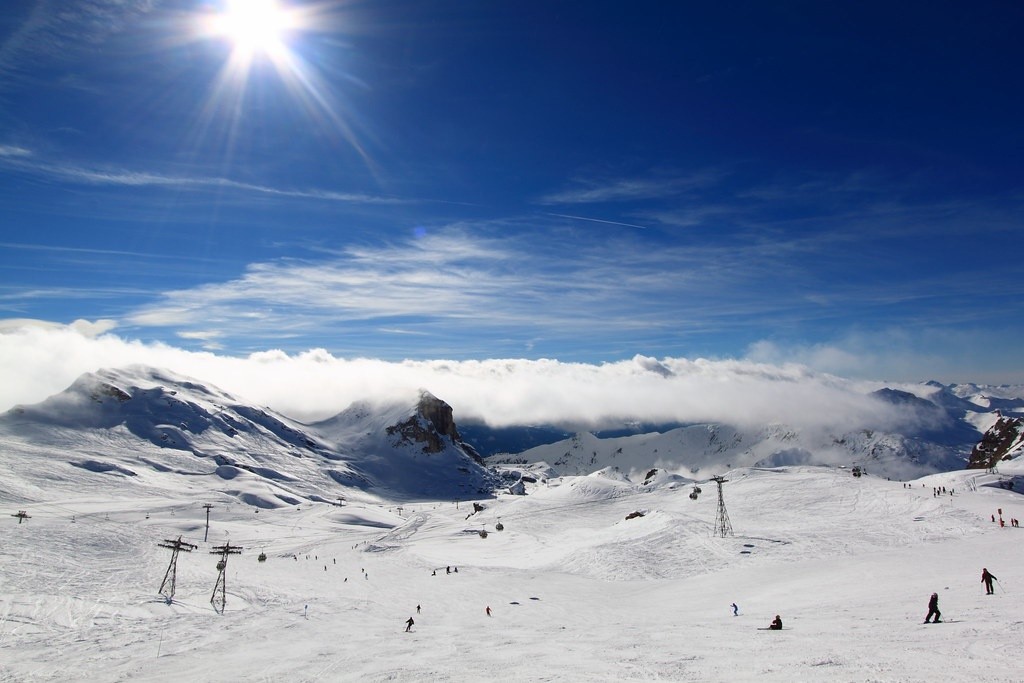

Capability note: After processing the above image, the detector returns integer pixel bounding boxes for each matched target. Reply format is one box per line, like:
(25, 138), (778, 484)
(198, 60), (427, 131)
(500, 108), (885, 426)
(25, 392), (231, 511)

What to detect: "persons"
(406, 617), (414, 632)
(730, 603), (738, 615)
(887, 476), (1019, 527)
(981, 568), (997, 595)
(925, 593), (941, 623)
(770, 616), (782, 630)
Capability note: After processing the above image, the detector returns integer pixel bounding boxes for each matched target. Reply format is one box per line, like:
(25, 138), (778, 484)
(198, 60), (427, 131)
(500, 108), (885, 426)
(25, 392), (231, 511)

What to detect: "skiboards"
(917, 620), (947, 624)
(733, 614), (744, 616)
(757, 626), (776, 631)
(403, 629), (412, 632)
(986, 592), (995, 596)
